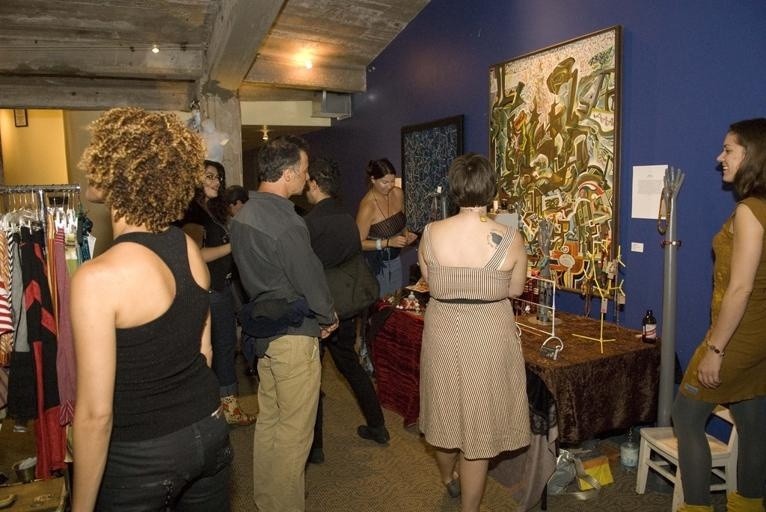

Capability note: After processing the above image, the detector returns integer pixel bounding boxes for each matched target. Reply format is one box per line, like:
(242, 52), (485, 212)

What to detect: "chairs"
(634, 404), (739, 511)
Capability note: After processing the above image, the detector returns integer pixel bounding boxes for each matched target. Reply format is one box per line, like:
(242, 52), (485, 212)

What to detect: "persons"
(173, 156), (256, 430)
(224, 184), (258, 379)
(356, 156), (418, 302)
(670, 118), (766, 512)
(226, 132), (338, 512)
(416, 151), (531, 511)
(64, 104), (237, 512)
(305, 151), (393, 464)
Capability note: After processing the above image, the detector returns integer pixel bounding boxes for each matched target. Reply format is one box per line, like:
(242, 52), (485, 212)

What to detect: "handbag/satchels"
(547, 448), (602, 501)
(324, 254), (380, 321)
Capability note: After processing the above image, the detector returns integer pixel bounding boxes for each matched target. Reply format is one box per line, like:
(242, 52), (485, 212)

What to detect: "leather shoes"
(358, 425), (389, 444)
(446, 471), (461, 497)
(307, 448), (324, 464)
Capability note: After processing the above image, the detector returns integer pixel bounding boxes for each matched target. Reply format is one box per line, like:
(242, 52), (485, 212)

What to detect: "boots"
(221, 394), (256, 427)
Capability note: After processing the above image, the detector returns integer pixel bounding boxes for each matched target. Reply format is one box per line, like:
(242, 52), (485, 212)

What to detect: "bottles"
(642, 309), (658, 344)
(620, 424), (640, 474)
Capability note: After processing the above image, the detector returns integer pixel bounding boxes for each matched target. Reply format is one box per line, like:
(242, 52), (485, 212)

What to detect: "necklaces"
(371, 186), (392, 228)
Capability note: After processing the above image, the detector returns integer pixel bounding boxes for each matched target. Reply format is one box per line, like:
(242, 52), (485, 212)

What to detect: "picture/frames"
(401, 114), (465, 240)
(487, 23), (625, 301)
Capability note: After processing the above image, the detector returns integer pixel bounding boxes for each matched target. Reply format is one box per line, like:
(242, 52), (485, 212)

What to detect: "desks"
(370, 295), (662, 446)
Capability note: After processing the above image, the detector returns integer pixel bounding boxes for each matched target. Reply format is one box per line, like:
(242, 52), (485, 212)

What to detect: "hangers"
(0, 184), (80, 236)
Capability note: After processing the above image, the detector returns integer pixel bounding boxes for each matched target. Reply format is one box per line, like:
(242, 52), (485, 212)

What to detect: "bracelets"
(705, 338), (728, 358)
(374, 238), (383, 251)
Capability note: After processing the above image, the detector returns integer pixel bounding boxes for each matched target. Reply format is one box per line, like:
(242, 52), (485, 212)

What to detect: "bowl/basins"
(11, 459), (36, 482)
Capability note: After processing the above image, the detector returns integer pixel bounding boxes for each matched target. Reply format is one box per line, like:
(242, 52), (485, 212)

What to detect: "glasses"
(206, 175), (223, 181)
